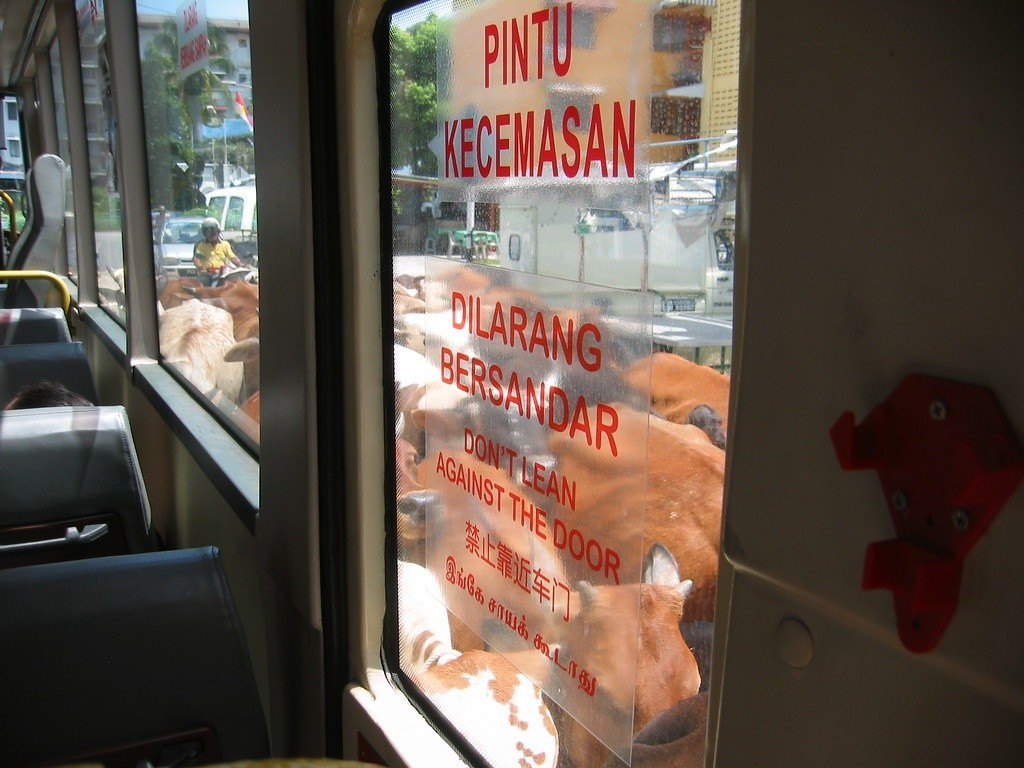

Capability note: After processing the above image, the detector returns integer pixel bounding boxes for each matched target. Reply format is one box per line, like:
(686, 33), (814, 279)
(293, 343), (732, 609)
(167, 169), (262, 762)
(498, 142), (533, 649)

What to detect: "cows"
(104, 267), (732, 768)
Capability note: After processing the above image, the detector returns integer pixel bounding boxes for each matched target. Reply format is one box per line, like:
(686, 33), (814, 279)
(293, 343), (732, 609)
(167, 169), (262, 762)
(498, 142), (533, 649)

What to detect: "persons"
(193, 218), (250, 276)
(5, 382), (164, 549)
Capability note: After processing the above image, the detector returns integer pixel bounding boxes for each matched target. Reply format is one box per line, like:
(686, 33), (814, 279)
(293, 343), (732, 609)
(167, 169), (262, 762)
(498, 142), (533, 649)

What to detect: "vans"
(158, 216), (204, 277)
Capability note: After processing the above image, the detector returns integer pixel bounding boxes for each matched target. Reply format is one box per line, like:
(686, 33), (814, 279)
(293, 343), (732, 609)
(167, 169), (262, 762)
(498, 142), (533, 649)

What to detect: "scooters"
(194, 250), (233, 286)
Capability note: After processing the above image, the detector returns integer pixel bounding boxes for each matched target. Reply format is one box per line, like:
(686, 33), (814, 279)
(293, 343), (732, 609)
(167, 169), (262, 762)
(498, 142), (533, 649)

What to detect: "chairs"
(0, 152), (274, 768)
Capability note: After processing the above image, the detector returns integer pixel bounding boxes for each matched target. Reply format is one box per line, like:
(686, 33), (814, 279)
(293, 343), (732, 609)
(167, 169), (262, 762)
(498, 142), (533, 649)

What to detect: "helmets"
(202, 218), (221, 233)
